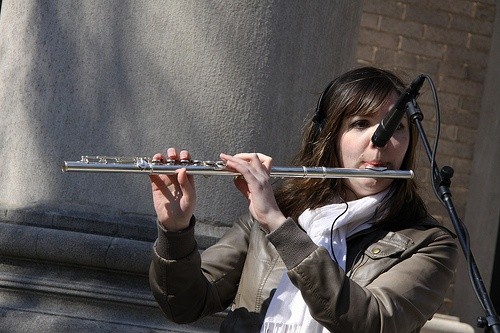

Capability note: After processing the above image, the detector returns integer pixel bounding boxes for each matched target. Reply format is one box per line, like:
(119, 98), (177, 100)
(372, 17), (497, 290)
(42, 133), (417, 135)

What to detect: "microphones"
(370, 74), (425, 148)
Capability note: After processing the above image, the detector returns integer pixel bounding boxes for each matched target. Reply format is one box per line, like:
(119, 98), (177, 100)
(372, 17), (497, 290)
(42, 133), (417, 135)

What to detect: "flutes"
(62, 155), (414, 179)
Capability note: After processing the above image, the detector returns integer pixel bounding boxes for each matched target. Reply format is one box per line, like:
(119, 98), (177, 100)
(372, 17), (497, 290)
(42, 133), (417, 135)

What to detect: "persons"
(147, 66), (458, 333)
